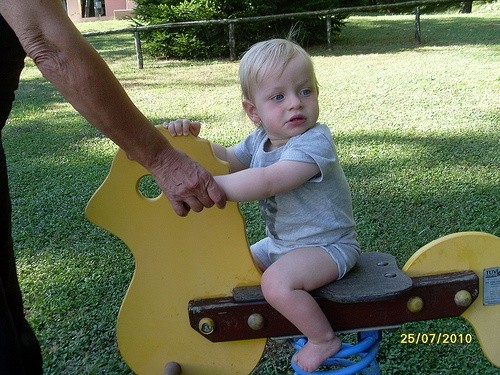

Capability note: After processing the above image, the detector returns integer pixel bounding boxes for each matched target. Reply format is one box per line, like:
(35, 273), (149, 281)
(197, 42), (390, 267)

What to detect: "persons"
(163, 23), (361, 371)
(1, 1), (227, 375)
